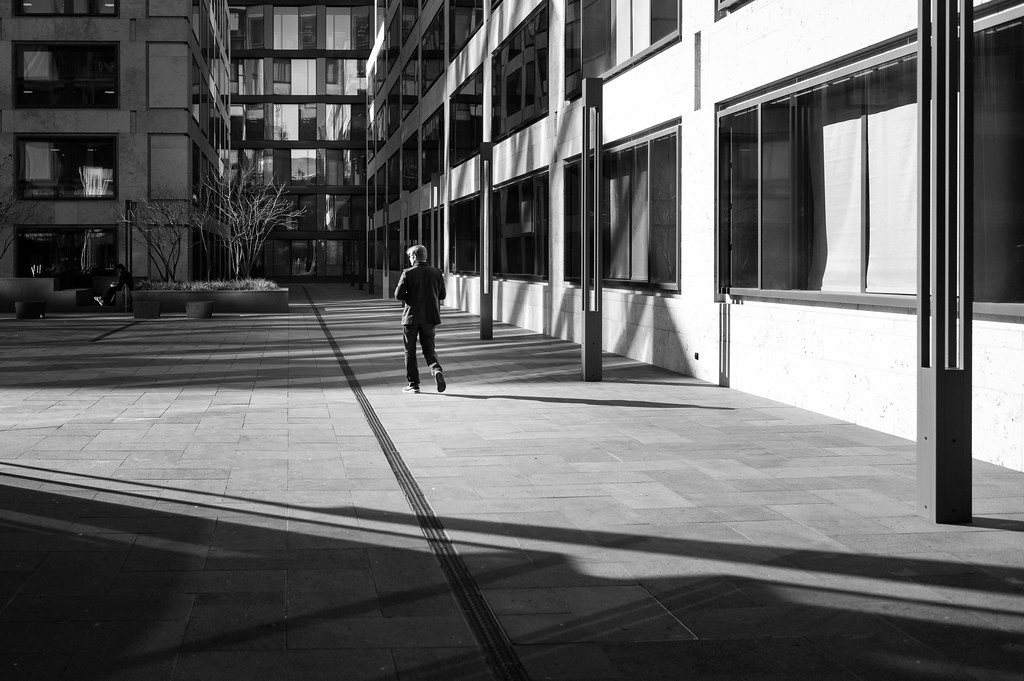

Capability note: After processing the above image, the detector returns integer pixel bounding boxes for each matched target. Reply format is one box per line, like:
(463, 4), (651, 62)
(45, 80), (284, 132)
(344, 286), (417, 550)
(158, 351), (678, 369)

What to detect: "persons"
(394, 245), (446, 393)
(93, 264), (133, 306)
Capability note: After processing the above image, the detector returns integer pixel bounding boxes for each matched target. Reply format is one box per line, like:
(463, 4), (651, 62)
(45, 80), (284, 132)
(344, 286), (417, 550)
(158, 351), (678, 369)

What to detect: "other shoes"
(435, 371), (447, 392)
(94, 296), (103, 307)
(402, 384), (420, 393)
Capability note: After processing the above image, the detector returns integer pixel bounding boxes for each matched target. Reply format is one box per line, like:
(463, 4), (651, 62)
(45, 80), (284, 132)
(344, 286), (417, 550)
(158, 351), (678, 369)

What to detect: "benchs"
(115, 291), (124, 307)
